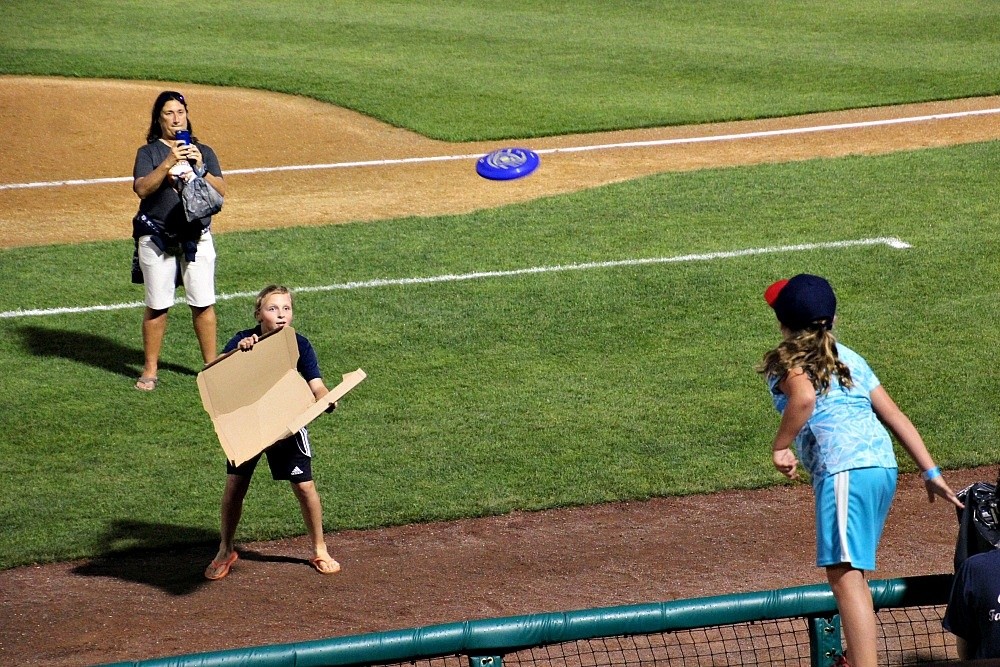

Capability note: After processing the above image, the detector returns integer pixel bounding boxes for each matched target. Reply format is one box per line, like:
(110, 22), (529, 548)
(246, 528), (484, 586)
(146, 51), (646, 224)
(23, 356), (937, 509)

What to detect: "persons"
(941, 475), (1000, 667)
(761, 273), (965, 667)
(202, 284), (342, 582)
(134, 91), (227, 391)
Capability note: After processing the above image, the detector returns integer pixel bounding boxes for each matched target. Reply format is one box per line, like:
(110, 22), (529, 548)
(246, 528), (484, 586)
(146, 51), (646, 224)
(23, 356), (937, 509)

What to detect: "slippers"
(308, 552), (340, 574)
(204, 551), (238, 580)
(134, 377), (157, 391)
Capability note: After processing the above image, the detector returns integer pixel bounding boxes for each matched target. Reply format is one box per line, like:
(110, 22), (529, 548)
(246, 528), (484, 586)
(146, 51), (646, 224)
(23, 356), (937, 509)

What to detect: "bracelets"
(920, 466), (941, 482)
(202, 169), (209, 178)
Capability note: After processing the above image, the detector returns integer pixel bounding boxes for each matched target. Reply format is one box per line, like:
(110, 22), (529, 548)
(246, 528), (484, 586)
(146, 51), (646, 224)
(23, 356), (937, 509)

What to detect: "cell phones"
(175, 130), (191, 157)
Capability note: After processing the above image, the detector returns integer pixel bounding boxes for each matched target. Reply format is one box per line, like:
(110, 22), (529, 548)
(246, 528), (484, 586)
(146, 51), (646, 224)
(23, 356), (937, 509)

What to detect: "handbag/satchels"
(181, 162), (223, 222)
(954, 482), (1000, 573)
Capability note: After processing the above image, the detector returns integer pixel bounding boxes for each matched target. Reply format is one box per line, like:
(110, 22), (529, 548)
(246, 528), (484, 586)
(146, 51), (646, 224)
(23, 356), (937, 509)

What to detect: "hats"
(764, 273), (836, 331)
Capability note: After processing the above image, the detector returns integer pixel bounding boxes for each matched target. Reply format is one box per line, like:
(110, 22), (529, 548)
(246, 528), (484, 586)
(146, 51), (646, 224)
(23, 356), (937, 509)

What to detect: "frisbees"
(475, 147), (539, 181)
